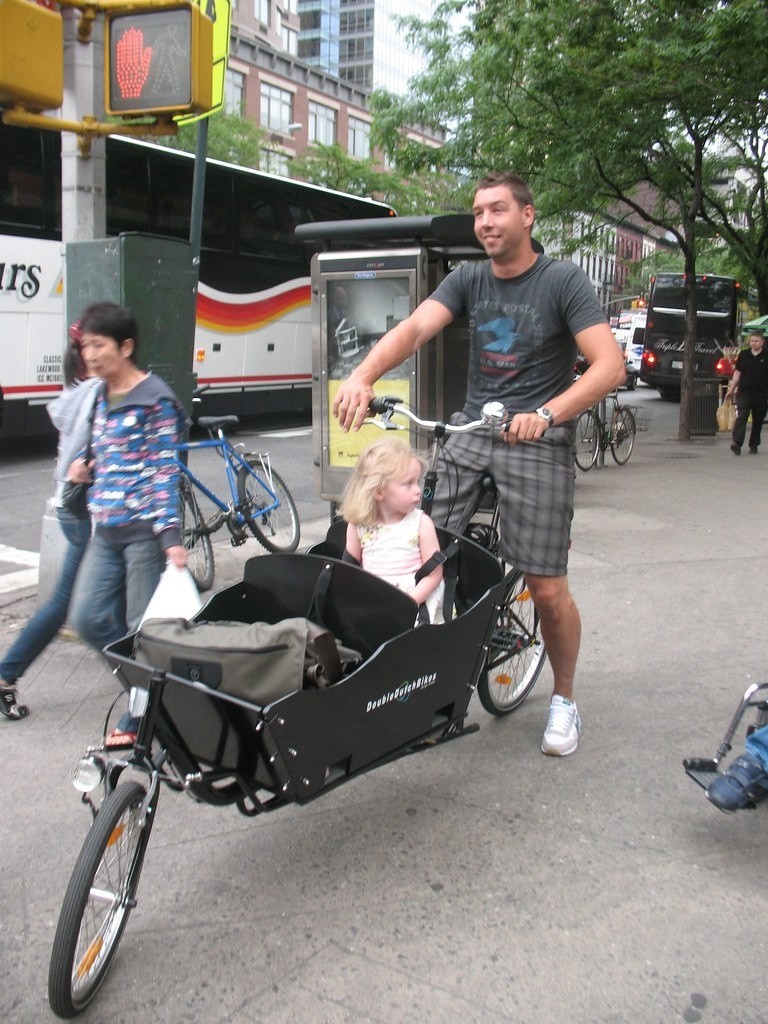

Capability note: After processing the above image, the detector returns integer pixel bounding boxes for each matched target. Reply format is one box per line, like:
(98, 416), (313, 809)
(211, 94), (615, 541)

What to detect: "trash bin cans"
(691, 378), (722, 435)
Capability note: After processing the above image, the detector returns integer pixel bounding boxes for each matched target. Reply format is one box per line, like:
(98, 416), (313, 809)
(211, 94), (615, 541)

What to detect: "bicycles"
(178, 416), (301, 593)
(46, 397), (571, 1021)
(574, 361), (654, 472)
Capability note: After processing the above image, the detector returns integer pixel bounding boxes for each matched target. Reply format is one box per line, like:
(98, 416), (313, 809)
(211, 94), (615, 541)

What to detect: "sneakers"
(542, 693), (582, 755)
(706, 754), (768, 811)
(0, 683), (29, 719)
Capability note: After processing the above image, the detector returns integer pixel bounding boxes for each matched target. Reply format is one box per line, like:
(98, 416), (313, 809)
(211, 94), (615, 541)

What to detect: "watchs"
(536, 407), (553, 428)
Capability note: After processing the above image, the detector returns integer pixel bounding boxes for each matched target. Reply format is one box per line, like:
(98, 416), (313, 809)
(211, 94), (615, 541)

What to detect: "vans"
(624, 312), (649, 374)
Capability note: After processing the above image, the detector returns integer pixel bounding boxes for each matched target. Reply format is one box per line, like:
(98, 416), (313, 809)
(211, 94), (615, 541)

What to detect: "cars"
(575, 355), (639, 391)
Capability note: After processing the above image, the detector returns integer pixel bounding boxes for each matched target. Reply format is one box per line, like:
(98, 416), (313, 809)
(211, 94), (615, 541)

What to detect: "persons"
(724, 331), (768, 455)
(0, 320), (109, 721)
(66, 301), (187, 752)
(331, 169), (627, 759)
(335, 441), (457, 625)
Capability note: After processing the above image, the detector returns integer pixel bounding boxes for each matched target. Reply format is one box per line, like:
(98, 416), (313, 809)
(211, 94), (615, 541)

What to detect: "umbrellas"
(741, 314), (768, 337)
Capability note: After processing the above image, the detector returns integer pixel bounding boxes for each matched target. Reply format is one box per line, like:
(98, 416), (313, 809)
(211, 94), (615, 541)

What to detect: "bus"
(637, 275), (745, 404)
(0, 108), (403, 439)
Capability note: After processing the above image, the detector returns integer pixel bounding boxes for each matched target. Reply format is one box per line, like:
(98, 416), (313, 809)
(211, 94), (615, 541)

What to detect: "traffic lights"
(104, 0), (212, 115)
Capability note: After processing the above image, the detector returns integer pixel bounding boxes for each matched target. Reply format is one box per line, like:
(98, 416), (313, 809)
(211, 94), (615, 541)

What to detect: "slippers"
(105, 728), (140, 750)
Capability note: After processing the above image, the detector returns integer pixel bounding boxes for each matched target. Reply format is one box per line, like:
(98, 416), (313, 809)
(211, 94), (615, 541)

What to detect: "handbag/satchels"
(127, 559), (204, 719)
(716, 398), (737, 432)
(62, 481), (92, 518)
(133, 616), (360, 706)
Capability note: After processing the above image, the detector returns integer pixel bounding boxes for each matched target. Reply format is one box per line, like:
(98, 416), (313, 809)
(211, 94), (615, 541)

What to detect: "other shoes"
(730, 443), (741, 455)
(749, 447), (758, 454)
